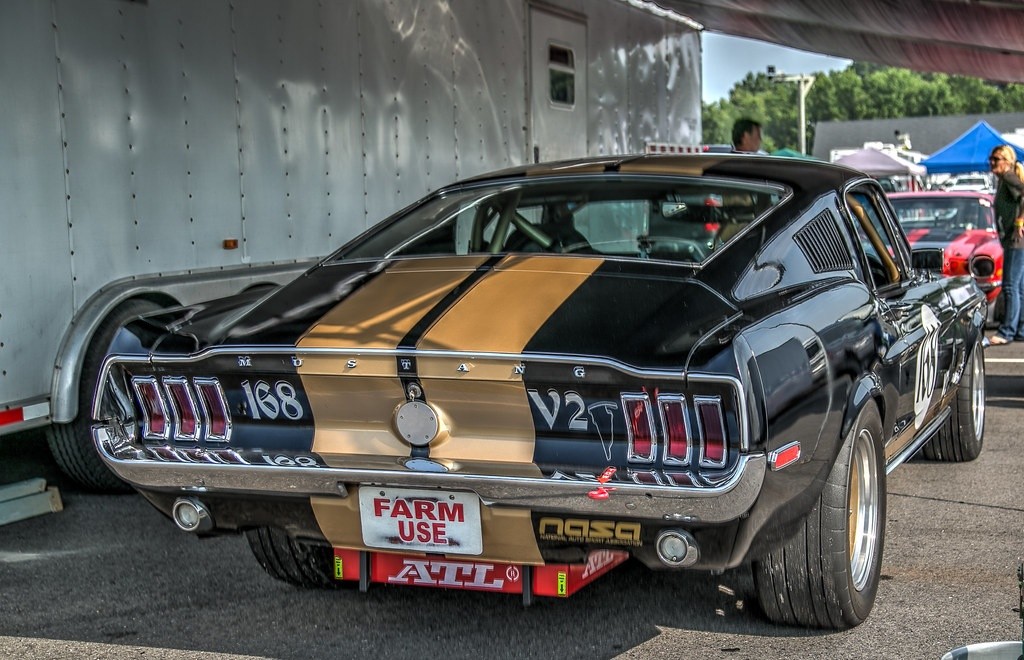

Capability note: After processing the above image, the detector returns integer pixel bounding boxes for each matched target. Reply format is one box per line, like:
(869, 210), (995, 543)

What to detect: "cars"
(853, 171), (1003, 328)
(86, 139), (994, 634)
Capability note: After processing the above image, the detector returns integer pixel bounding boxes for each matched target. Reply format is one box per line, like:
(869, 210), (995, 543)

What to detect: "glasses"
(988, 156), (1005, 162)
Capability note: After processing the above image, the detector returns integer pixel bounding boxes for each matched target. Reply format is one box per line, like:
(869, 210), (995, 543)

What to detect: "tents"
(769, 119), (1024, 192)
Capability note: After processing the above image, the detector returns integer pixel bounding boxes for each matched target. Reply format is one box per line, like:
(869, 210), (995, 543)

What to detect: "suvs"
(635, 145), (786, 265)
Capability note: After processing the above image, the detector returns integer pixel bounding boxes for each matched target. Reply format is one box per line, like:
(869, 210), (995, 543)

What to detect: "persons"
(989, 144), (1024, 346)
(732, 117), (761, 155)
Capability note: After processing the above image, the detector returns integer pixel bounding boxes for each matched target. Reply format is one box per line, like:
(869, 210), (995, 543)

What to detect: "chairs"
(504, 223), (592, 254)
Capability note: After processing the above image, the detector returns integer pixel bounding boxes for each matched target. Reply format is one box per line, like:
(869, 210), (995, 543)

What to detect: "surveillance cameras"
(896, 132), (910, 141)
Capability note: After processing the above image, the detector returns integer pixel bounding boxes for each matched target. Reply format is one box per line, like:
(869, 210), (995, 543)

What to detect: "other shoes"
(990, 334), (1009, 345)
